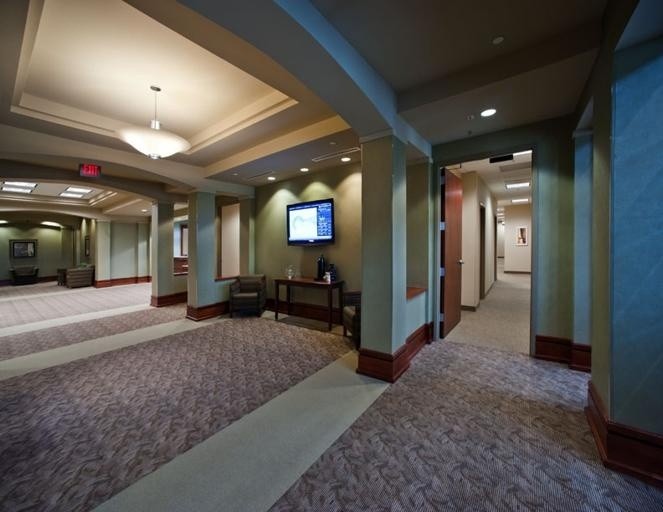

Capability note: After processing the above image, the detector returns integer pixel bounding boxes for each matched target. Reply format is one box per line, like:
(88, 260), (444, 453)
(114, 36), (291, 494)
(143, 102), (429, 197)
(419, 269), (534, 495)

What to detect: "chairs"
(229, 274), (266, 318)
(343, 288), (360, 350)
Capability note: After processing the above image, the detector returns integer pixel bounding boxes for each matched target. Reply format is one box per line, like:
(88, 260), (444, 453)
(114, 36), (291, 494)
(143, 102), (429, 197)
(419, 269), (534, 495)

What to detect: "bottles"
(327, 263), (337, 281)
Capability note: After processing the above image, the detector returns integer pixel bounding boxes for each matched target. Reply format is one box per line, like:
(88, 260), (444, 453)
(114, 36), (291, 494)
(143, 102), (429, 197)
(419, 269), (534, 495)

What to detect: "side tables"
(57, 268), (67, 286)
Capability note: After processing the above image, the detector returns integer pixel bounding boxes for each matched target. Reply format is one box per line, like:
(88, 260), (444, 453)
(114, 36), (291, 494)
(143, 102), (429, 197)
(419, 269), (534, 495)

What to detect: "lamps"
(112, 86), (192, 160)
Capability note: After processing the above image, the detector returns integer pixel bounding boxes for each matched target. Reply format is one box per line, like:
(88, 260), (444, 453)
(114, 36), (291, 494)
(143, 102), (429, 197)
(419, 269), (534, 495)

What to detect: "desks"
(273, 276), (345, 331)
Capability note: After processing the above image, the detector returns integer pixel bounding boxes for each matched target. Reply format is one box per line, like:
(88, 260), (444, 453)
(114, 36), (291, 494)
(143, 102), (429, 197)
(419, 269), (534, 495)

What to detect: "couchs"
(7, 265), (39, 285)
(66, 264), (95, 288)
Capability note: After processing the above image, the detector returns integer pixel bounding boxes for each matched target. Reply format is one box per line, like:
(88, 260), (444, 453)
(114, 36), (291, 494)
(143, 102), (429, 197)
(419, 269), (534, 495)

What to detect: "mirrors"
(516, 227), (527, 245)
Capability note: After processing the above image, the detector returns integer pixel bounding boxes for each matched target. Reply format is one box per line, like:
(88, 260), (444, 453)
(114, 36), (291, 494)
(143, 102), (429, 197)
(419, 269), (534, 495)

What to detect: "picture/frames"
(9, 238), (38, 260)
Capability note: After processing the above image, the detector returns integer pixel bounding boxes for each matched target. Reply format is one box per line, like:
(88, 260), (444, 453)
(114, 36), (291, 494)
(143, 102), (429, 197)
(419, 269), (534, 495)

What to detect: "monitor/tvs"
(12, 241), (35, 258)
(287, 198), (335, 247)
